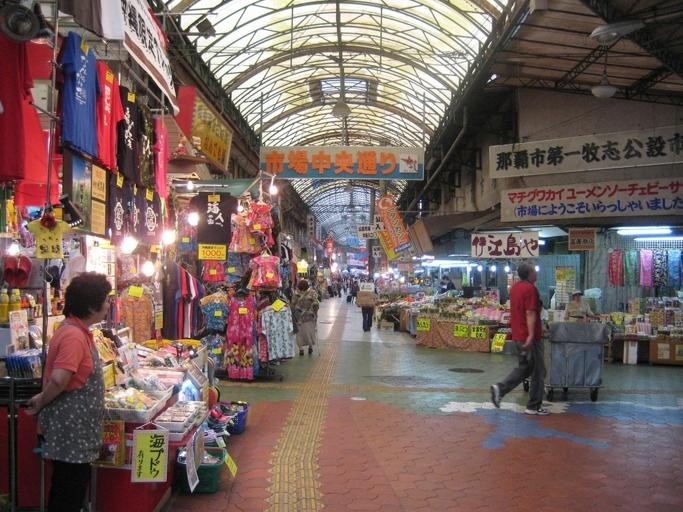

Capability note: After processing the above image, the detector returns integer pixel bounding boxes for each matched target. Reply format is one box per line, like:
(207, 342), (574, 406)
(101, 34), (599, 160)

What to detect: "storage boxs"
(357, 283), (376, 306)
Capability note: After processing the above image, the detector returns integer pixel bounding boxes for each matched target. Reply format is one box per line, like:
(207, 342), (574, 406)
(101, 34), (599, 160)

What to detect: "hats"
(572, 290), (582, 296)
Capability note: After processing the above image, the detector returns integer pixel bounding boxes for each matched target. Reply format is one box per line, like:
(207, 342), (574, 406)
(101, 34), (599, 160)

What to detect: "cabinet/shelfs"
(1, 376), (210, 511)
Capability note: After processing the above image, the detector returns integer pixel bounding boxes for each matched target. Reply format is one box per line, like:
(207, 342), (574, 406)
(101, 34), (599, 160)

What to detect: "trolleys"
(523, 321), (609, 402)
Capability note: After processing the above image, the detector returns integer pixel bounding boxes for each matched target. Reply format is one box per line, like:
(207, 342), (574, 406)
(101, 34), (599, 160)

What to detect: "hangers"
(253, 244), (280, 262)
(233, 194), (272, 219)
(207, 287), (290, 313)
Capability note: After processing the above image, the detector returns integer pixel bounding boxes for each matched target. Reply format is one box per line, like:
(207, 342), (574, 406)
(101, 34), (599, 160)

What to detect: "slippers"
(4, 254), (33, 289)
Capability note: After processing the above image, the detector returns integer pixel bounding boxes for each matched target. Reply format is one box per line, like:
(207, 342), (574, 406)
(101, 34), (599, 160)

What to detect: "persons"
(563, 289), (595, 322)
(24, 271), (111, 512)
(504, 285), (512, 303)
(327, 274), (376, 332)
(441, 275), (456, 293)
(290, 280), (320, 356)
(490, 262), (551, 415)
(539, 300), (549, 320)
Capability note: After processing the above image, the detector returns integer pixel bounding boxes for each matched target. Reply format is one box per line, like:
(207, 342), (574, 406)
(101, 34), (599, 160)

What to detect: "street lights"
(590, 10), (683, 45)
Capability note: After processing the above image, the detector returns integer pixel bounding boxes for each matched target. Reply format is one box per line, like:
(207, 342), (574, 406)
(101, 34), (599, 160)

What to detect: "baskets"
(215, 401), (249, 435)
(177, 446), (226, 494)
(104, 380), (210, 442)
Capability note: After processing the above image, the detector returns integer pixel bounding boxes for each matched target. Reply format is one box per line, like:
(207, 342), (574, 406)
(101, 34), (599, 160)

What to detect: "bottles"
(0, 288), (22, 324)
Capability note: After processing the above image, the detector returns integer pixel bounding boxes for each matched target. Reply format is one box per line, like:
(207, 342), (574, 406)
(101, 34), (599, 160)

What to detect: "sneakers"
(491, 384), (501, 408)
(524, 407), (551, 416)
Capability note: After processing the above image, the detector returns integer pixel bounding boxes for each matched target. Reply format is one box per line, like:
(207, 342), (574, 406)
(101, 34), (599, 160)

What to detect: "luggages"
(347, 296), (352, 302)
